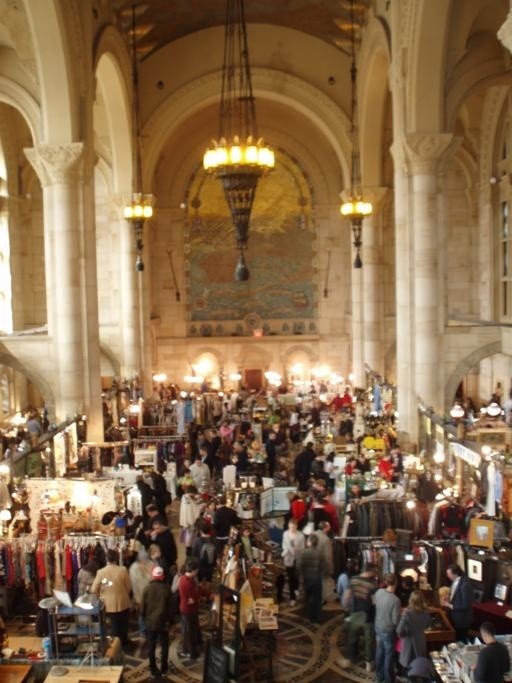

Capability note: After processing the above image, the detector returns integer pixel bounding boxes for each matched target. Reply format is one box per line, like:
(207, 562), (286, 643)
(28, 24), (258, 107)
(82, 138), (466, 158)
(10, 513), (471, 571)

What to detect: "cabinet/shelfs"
(47, 600), (105, 657)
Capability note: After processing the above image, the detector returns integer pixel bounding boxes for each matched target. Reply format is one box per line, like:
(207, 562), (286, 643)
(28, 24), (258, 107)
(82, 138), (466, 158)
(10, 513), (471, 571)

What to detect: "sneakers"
(150, 639), (204, 677)
(290, 589), (300, 606)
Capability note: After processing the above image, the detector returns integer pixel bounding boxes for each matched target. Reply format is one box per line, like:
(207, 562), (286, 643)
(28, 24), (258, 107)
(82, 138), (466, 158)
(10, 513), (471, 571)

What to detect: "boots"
(367, 661), (374, 672)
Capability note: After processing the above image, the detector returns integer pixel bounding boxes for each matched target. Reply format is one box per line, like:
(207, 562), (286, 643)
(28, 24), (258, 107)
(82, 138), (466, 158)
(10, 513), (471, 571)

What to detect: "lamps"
(203, 0), (277, 283)
(339, 0), (373, 269)
(122, 4), (155, 270)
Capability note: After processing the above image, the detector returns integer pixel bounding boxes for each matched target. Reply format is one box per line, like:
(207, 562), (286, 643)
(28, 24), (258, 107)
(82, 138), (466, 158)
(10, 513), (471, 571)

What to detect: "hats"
(152, 565), (164, 576)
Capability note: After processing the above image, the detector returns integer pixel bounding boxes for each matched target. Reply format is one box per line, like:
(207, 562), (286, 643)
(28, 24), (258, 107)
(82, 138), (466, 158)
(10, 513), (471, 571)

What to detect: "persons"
(2, 371), (512, 682)
